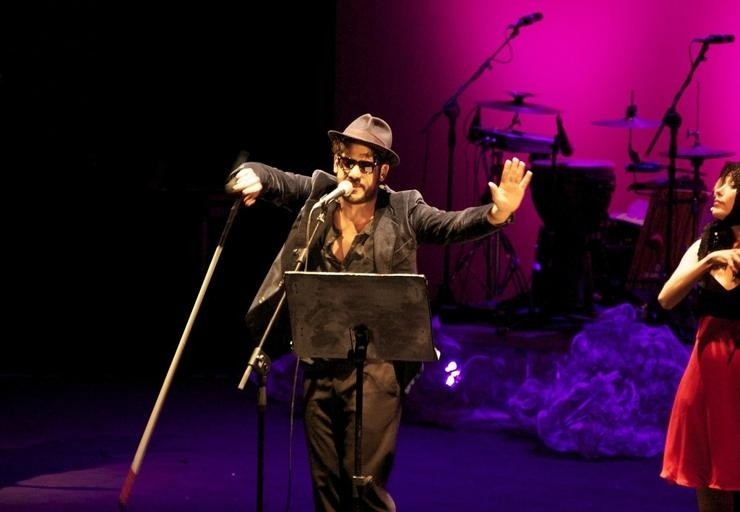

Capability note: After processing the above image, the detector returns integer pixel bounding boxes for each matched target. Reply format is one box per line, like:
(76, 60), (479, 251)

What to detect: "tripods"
(449, 150), (529, 303)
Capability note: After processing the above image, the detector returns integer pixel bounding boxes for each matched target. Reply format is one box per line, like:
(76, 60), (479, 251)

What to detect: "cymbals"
(657, 147), (735, 158)
(592, 118), (665, 129)
(474, 101), (560, 115)
(466, 128), (556, 154)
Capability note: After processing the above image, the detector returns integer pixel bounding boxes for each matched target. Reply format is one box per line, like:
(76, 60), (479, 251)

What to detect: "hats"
(327, 114), (401, 166)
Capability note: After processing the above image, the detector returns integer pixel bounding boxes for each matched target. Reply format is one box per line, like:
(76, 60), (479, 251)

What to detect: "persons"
(227, 114), (533, 512)
(657, 161), (740, 512)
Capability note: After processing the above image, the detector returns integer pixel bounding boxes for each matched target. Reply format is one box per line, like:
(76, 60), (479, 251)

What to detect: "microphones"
(519, 12), (541, 26)
(320, 179), (356, 208)
(705, 34), (736, 43)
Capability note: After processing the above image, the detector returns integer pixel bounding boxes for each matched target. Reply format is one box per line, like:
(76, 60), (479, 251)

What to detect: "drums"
(530, 160), (613, 229)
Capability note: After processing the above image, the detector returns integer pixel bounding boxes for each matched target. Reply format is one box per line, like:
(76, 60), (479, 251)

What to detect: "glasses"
(337, 154), (378, 174)
